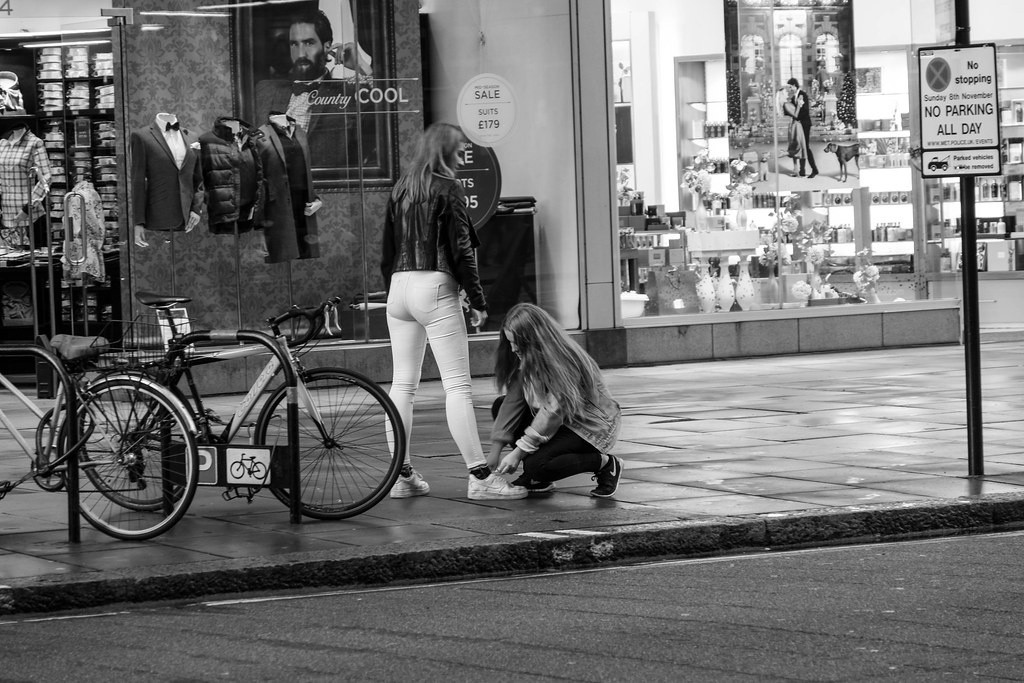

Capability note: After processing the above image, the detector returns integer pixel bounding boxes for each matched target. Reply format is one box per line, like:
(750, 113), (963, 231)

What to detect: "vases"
(621, 153), (909, 312)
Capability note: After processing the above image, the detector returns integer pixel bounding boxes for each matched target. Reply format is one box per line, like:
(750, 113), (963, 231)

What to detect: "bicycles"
(77, 289), (408, 524)
(0, 330), (202, 547)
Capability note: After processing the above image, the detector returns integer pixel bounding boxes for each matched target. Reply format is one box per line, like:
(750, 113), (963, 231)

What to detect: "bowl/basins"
(620, 293), (649, 318)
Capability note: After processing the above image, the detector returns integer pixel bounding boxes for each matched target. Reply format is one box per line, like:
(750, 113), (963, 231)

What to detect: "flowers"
(618, 138), (911, 288)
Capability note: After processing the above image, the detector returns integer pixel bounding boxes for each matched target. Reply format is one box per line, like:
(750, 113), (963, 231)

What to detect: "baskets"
(90, 313), (199, 368)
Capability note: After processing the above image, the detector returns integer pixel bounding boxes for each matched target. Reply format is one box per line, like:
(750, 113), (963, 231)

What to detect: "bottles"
(927, 174), (1009, 236)
(751, 191), (775, 208)
(686, 261), (756, 314)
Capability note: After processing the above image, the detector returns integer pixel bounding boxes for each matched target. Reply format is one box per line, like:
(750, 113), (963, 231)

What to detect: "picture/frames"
(229, 0), (400, 195)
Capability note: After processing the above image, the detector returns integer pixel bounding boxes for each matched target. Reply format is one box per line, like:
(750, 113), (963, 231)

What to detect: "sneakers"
(590, 453), (623, 497)
(468, 469), (527, 499)
(510, 472), (556, 491)
(389, 467), (430, 498)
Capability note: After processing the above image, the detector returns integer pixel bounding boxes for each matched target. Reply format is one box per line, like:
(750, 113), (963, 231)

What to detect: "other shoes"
(808, 171), (818, 178)
(799, 169), (805, 177)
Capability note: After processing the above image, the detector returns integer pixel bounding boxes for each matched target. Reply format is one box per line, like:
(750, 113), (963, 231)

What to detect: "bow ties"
(293, 82), (319, 96)
(166, 121), (181, 131)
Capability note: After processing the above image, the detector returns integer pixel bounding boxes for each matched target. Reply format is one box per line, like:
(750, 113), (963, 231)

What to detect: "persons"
(485, 302), (624, 497)
(60, 182), (105, 282)
(382, 124), (530, 500)
(255, 110), (323, 265)
(255, 7), (375, 166)
(778, 79), (819, 179)
(198, 115), (264, 235)
(126, 112), (206, 247)
(0, 124), (52, 251)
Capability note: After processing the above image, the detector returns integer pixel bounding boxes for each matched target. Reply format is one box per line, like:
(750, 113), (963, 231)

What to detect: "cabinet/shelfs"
(0, 39), (122, 349)
(692, 86), (1024, 321)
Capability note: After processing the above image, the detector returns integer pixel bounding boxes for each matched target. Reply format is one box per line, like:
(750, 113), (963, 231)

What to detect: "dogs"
(824, 143), (860, 182)
(759, 152), (771, 182)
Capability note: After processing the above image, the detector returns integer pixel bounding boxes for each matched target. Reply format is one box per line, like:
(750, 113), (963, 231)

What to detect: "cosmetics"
(619, 99), (1024, 248)
